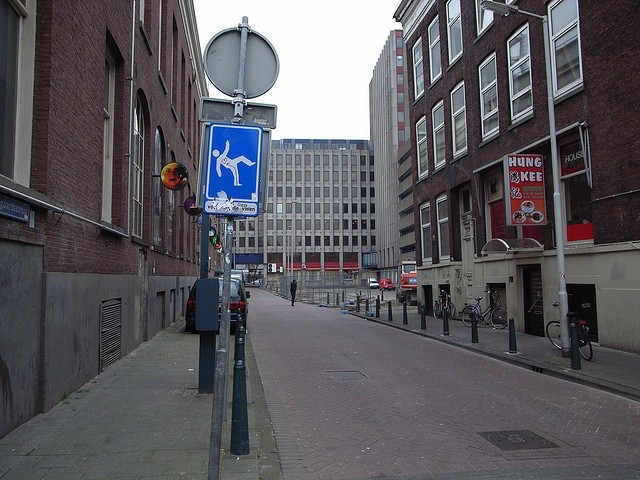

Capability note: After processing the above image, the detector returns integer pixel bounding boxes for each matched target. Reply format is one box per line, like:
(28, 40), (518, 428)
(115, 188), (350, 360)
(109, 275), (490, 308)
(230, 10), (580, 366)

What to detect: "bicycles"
(462, 289), (510, 330)
(433, 290), (456, 321)
(546, 300), (593, 361)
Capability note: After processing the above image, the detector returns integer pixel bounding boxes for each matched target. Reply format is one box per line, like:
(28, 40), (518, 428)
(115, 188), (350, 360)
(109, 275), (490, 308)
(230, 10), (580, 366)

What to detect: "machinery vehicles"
(396, 259), (417, 305)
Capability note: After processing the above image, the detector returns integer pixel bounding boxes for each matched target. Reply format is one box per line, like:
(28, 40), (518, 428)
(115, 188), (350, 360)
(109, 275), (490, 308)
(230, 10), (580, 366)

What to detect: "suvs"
(378, 278), (396, 292)
(366, 277), (379, 289)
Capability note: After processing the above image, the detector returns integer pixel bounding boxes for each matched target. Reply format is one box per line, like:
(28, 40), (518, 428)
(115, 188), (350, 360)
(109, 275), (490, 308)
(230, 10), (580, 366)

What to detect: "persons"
(290, 280), (298, 306)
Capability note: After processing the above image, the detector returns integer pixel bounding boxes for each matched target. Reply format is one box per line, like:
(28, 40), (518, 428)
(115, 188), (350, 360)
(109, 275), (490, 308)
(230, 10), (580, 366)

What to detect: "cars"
(185, 278), (251, 334)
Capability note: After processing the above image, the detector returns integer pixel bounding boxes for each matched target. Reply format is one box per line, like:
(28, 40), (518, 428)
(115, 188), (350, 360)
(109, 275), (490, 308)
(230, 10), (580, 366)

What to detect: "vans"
(213, 269), (246, 288)
